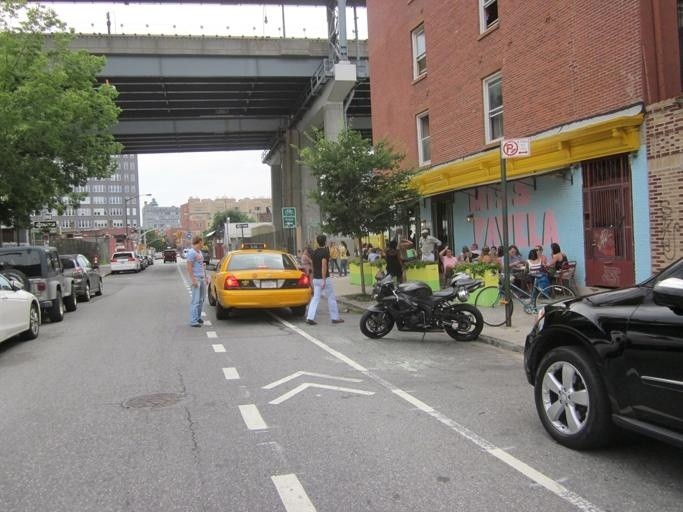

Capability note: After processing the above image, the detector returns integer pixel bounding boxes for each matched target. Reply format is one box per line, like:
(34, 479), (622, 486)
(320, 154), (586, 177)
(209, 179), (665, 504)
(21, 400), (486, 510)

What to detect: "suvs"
(0, 243), (76, 322)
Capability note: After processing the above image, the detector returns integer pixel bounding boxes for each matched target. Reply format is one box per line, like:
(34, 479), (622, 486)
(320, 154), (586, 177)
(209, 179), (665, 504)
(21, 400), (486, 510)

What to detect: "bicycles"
(474, 262), (577, 328)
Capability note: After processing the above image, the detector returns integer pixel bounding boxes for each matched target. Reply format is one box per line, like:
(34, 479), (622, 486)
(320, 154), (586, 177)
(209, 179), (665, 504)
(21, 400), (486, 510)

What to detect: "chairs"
(442, 256), (577, 296)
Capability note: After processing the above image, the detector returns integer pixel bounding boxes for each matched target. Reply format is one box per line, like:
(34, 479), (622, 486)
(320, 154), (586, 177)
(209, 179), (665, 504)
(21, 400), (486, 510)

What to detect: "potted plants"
(346, 256), (371, 286)
(450, 261), (500, 306)
(369, 258), (397, 286)
(400, 260), (441, 292)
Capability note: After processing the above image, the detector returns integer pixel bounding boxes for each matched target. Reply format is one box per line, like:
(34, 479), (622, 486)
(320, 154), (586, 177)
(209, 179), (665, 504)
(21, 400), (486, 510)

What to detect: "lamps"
(464, 213), (474, 224)
(569, 162), (578, 175)
(420, 218), (426, 227)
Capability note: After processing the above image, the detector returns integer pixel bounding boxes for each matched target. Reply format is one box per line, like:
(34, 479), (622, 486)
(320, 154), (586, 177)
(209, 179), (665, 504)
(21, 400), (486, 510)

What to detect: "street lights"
(124, 193), (153, 250)
(141, 203), (159, 255)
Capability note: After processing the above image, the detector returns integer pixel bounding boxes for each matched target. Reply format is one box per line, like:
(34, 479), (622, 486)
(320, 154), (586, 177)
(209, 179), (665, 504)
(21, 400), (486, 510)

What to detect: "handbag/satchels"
(346, 249), (350, 256)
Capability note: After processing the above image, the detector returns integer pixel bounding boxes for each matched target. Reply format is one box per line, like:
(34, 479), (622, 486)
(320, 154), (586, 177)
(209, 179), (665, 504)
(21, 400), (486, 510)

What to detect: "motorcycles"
(360, 266), (486, 343)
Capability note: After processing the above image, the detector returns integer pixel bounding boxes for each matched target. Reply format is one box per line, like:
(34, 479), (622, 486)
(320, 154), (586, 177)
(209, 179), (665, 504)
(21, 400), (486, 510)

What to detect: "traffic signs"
(282, 207), (297, 228)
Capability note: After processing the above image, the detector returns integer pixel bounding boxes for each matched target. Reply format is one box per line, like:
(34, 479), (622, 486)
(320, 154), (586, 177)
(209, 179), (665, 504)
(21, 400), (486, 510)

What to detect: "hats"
(421, 230), (429, 234)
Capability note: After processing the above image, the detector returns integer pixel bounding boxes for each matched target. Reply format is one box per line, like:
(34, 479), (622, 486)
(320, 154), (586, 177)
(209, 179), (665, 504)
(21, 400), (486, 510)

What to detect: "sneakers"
(191, 319), (203, 327)
(332, 318), (344, 323)
(306, 319), (317, 324)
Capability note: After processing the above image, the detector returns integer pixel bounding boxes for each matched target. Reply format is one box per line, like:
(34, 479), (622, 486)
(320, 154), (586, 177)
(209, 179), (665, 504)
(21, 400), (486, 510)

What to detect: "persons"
(298, 248), (314, 295)
(92, 255), (100, 274)
(306, 235), (345, 324)
(459, 244), (568, 298)
(338, 241), (349, 276)
(419, 229), (442, 262)
(187, 237), (209, 327)
(439, 245), (458, 289)
(354, 240), (418, 261)
(328, 241), (340, 273)
(385, 241), (403, 284)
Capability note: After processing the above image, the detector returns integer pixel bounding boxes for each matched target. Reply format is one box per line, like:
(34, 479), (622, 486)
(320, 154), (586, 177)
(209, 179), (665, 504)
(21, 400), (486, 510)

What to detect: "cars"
(109, 246), (210, 275)
(522, 257), (682, 453)
(0, 273), (42, 345)
(56, 252), (104, 303)
(207, 248), (314, 321)
